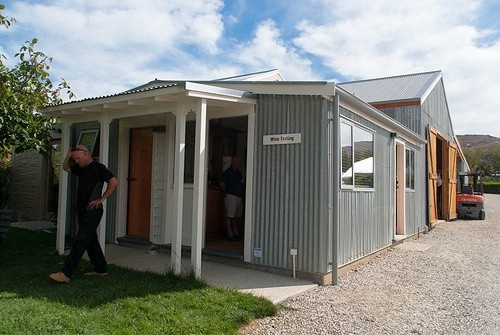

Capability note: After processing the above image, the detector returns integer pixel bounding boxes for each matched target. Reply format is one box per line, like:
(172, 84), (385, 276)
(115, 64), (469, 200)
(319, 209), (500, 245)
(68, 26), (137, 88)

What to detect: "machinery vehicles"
(457, 172), (486, 220)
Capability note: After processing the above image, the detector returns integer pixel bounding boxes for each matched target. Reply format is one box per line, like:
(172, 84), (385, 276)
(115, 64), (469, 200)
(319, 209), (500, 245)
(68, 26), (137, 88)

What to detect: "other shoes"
(83, 270), (109, 277)
(226, 234), (241, 241)
(49, 270), (71, 283)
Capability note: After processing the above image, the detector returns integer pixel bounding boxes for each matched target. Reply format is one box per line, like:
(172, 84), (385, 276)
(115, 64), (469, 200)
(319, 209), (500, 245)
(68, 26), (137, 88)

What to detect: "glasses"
(71, 147), (89, 152)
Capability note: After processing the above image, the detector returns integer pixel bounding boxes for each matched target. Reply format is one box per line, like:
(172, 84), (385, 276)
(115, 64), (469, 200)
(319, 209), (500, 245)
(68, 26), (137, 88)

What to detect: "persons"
(49, 144), (118, 283)
(220, 156), (246, 241)
